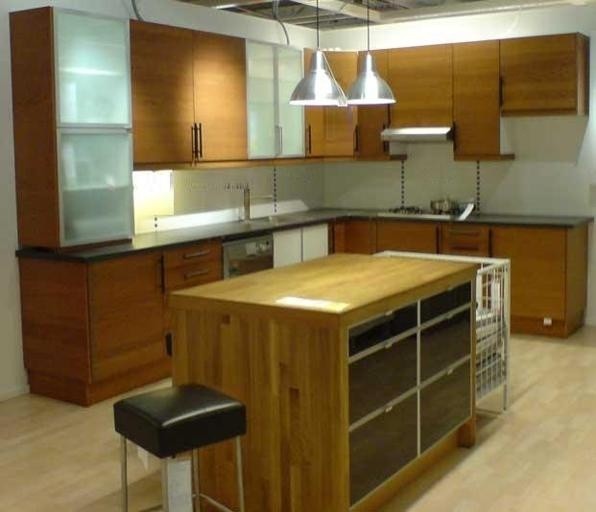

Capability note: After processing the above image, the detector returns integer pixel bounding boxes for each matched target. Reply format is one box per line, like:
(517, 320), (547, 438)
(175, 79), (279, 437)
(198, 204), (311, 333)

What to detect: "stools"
(114, 382), (247, 512)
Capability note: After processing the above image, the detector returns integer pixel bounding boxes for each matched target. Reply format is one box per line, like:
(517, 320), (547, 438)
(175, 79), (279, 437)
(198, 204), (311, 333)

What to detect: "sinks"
(254, 214), (291, 225)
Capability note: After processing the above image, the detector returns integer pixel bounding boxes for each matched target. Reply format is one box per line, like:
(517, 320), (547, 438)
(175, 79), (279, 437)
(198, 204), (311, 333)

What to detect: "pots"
(430, 198), (475, 212)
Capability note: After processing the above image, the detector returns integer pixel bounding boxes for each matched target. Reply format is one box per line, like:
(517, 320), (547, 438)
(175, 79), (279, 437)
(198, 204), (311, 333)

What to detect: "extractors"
(379, 123), (454, 143)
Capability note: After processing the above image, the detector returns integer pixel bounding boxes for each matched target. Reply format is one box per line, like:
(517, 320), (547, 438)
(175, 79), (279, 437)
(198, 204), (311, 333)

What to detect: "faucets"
(242, 185), (251, 223)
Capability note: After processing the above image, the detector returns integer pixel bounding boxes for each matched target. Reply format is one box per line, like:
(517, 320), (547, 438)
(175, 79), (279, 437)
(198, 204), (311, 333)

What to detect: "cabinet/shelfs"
(8, 5), (135, 249)
(330, 222), (590, 337)
(130, 18), (307, 172)
(167, 252), (483, 511)
(305, 33), (590, 163)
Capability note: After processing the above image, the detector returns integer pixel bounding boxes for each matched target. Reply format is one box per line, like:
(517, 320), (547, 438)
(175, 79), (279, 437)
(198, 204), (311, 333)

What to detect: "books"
(475, 308), (506, 394)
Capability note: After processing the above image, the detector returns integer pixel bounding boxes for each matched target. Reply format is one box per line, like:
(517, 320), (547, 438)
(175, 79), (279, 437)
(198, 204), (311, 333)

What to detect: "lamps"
(287, 1), (397, 106)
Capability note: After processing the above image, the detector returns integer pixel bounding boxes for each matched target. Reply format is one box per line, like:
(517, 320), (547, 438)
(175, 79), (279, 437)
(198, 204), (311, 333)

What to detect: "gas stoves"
(377, 206), (466, 221)
